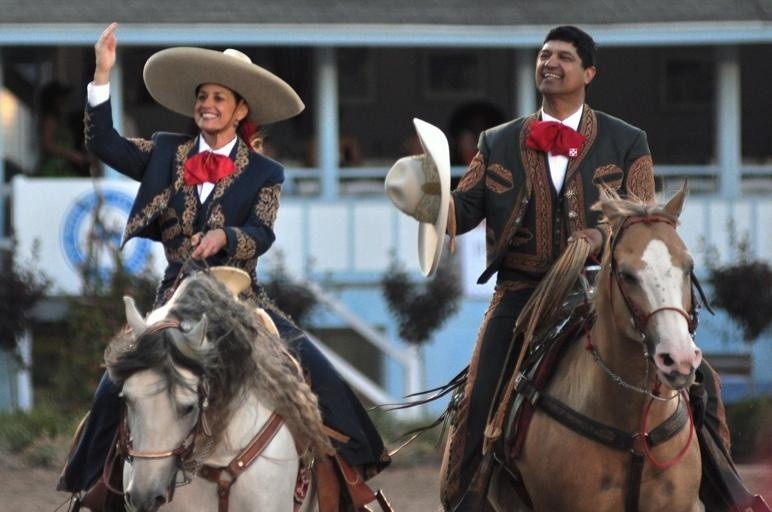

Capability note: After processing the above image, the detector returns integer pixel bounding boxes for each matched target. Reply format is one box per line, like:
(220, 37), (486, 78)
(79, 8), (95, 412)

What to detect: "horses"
(364, 175), (706, 512)
(98, 246), (342, 512)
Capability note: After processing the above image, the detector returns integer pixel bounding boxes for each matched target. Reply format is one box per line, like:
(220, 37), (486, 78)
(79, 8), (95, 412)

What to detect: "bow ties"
(525, 121), (586, 158)
(183, 152), (237, 186)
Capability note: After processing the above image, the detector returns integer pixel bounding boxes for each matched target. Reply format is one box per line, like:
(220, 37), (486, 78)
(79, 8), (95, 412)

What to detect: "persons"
(53, 23), (391, 494)
(441, 26), (771, 510)
(450, 124), (477, 167)
(308, 131), (359, 169)
(33, 80), (84, 180)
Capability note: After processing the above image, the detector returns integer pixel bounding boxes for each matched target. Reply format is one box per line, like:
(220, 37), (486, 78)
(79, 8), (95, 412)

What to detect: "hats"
(143, 45), (305, 125)
(385, 117), (451, 277)
